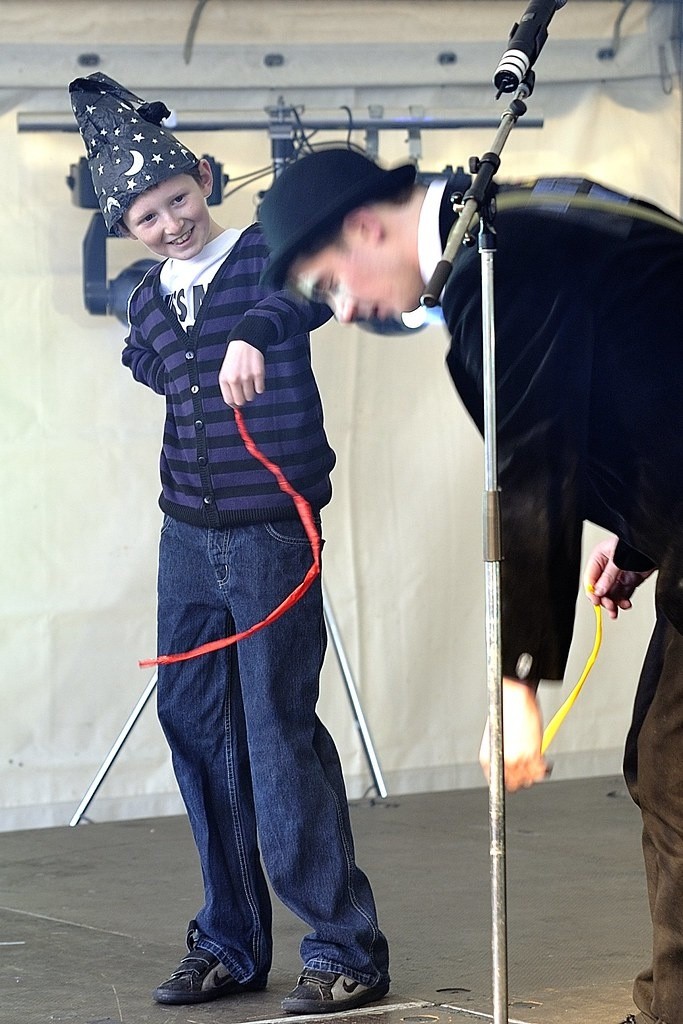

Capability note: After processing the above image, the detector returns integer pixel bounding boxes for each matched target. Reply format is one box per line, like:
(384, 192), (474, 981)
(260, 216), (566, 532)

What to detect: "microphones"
(492, 0), (568, 101)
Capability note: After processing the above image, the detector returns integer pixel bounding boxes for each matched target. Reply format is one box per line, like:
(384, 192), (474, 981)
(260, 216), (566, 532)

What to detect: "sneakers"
(153, 947), (268, 1004)
(281, 969), (390, 1013)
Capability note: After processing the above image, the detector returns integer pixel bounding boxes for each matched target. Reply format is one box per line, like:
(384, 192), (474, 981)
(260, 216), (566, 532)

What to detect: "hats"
(68, 72), (200, 234)
(257, 148), (413, 287)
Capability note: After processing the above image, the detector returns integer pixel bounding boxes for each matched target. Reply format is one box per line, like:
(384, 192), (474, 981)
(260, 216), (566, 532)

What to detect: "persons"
(256, 148), (683, 1024)
(67, 72), (389, 1013)
